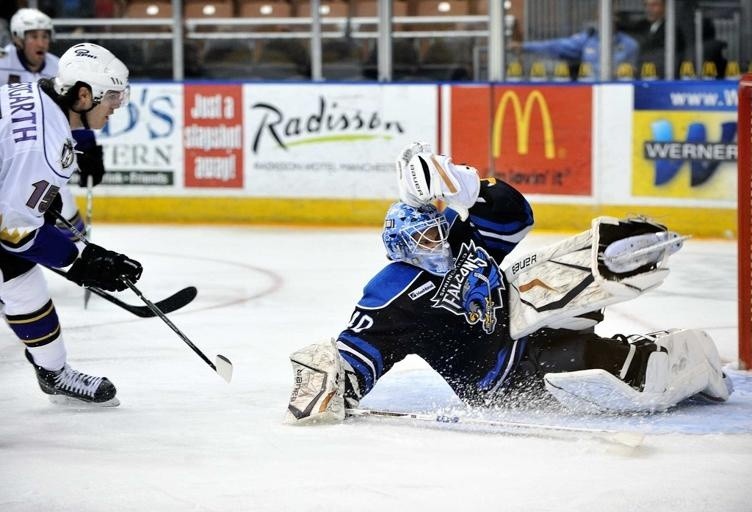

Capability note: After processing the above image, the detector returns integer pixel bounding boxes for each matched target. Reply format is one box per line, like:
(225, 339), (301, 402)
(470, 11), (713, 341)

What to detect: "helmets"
(53, 41), (129, 104)
(10, 7), (56, 41)
(382, 198), (457, 277)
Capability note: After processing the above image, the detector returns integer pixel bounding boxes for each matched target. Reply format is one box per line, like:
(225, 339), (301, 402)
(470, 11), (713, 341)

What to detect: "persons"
(588, 0), (686, 80)
(287, 139), (736, 426)
(509, 8), (641, 79)
(0, 7), (89, 259)
(1, 42), (143, 405)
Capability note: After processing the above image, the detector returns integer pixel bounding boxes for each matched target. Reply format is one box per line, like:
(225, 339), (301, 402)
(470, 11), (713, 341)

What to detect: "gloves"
(76, 144), (106, 188)
(396, 142), (481, 210)
(285, 339), (362, 419)
(79, 240), (143, 292)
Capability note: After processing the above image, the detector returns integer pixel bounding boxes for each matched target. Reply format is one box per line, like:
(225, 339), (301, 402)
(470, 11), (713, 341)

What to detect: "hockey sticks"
(55, 211), (232, 382)
(41, 266), (198, 318)
(344, 407), (660, 445)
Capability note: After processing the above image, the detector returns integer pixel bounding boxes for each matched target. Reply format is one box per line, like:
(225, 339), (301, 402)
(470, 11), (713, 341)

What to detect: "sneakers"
(600, 231), (684, 275)
(23, 346), (116, 403)
